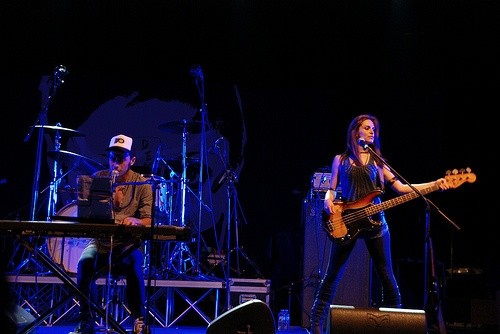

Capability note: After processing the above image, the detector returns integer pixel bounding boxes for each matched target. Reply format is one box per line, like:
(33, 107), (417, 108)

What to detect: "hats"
(104, 134), (135, 156)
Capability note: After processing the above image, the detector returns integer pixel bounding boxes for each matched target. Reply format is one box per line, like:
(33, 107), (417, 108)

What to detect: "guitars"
(321, 167), (478, 245)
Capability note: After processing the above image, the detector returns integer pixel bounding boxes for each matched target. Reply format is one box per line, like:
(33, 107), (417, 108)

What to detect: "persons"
(310, 114), (449, 334)
(70, 134), (152, 334)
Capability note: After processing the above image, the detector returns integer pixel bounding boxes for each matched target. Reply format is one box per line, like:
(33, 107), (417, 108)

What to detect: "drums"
(141, 174), (173, 219)
(47, 199), (96, 276)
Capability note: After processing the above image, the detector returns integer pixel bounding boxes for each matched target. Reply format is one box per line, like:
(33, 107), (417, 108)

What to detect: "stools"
(89, 261), (135, 334)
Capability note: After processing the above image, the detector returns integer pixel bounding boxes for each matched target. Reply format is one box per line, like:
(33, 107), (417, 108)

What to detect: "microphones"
(357, 137), (367, 150)
(186, 68), (205, 80)
(59, 65), (69, 75)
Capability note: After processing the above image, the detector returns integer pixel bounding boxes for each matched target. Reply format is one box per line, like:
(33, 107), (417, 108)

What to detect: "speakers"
(325, 304), (427, 334)
(300, 198), (372, 334)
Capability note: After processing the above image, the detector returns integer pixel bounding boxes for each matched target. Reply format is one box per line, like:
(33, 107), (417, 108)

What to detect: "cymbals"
(177, 149), (216, 162)
(157, 119), (208, 134)
(27, 123), (82, 135)
(45, 149), (105, 173)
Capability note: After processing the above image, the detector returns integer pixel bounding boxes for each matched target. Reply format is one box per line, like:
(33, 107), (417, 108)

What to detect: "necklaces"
(361, 152), (368, 153)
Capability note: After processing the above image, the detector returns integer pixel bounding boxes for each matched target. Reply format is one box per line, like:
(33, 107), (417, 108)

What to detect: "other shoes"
(68, 322), (96, 334)
(133, 317), (145, 334)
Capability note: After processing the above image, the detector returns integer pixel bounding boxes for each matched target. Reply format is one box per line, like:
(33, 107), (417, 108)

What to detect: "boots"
(383, 287), (402, 308)
(310, 273), (338, 334)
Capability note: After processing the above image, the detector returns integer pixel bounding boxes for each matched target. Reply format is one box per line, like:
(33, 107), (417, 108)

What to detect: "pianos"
(0, 219), (195, 334)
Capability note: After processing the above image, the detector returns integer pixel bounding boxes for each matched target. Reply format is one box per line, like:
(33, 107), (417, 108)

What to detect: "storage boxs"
(227, 277), (271, 315)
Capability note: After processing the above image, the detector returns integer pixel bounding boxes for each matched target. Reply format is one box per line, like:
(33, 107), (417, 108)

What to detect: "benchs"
(7, 275), (223, 325)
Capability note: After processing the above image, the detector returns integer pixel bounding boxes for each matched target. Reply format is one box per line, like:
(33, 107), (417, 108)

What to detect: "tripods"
(10, 76), (264, 278)
